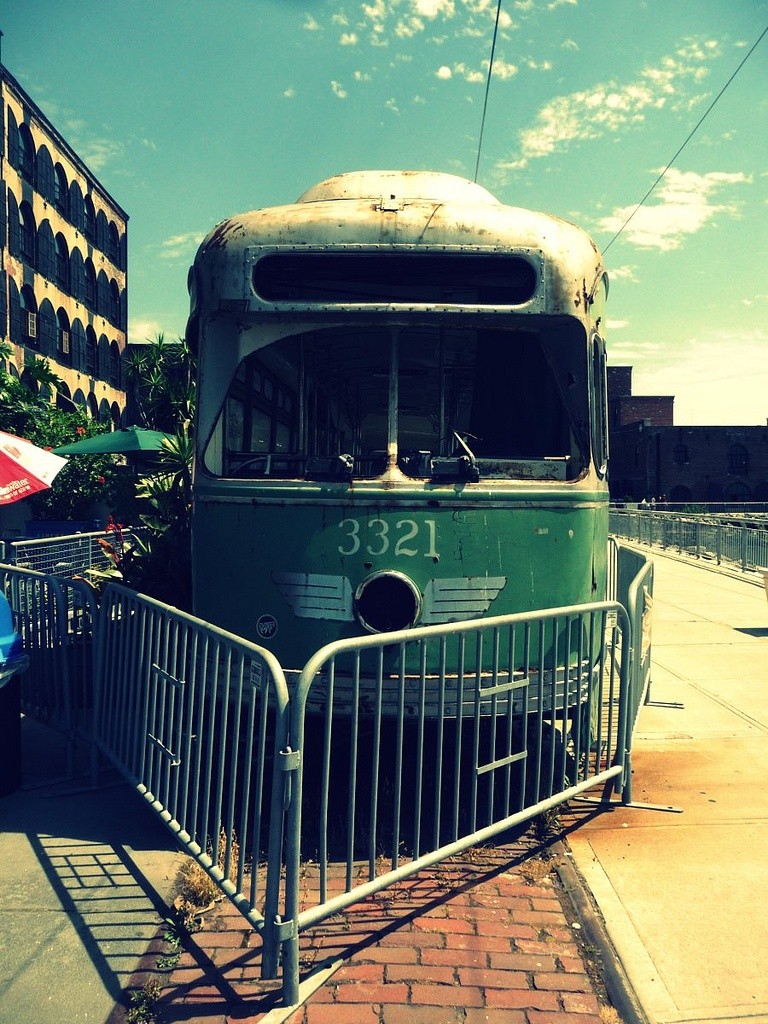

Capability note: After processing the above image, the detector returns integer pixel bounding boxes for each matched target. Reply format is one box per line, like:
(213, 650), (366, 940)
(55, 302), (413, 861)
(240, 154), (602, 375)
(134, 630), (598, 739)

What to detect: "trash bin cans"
(0, 587), (31, 800)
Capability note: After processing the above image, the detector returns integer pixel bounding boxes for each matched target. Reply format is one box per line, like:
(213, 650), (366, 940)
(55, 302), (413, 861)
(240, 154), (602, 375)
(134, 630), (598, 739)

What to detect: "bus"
(183, 169), (618, 730)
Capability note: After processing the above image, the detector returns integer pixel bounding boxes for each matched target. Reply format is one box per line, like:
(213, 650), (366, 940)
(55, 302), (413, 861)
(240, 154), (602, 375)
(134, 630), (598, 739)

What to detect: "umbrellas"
(51, 425), (190, 455)
(1, 430), (69, 504)
